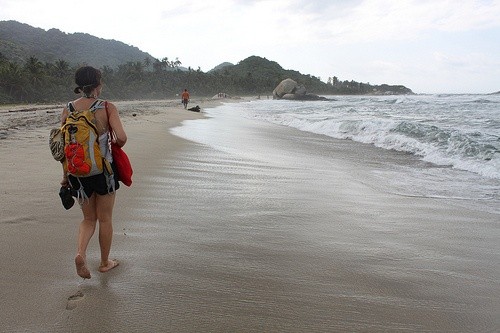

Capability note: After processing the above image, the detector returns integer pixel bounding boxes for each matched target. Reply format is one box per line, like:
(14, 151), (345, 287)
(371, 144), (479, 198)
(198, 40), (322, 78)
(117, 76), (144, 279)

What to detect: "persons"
(218, 91), (226, 99)
(60, 66), (127, 279)
(181, 89), (190, 109)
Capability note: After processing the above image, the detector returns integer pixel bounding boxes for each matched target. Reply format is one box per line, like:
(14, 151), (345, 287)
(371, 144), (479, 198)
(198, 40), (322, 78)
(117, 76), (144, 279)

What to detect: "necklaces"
(81, 93), (97, 98)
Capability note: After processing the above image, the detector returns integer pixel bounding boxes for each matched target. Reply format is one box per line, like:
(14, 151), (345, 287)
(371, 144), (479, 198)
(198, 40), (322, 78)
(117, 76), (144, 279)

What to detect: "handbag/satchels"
(105, 102), (133, 187)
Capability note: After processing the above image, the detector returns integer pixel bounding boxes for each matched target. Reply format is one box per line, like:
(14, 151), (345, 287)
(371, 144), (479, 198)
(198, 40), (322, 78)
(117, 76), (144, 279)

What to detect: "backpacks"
(59, 99), (114, 178)
